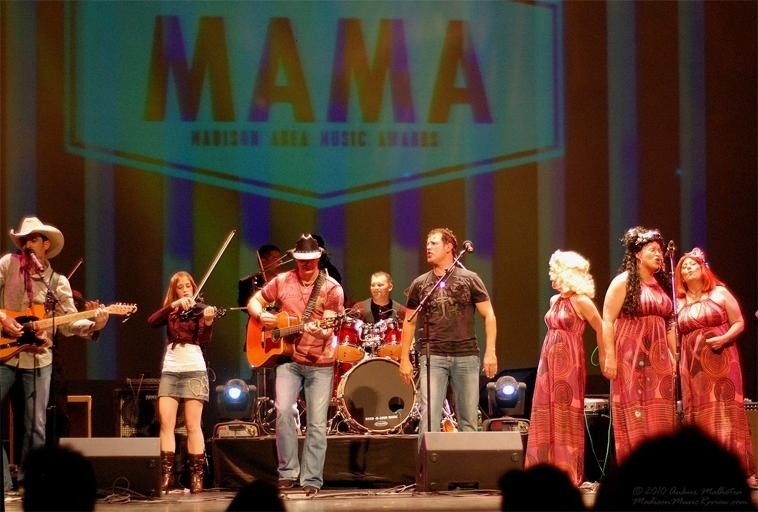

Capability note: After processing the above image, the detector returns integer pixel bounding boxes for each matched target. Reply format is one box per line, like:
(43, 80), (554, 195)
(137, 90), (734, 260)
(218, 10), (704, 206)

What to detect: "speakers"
(416, 431), (524, 491)
(113, 384), (184, 438)
(59, 437), (161, 496)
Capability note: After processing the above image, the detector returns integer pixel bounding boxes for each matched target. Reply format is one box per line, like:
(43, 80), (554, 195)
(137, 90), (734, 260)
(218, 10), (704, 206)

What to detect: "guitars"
(246, 310), (360, 368)
(0, 302), (139, 362)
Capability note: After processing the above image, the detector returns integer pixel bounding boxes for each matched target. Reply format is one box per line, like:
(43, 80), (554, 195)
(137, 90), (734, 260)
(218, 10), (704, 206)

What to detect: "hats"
(9, 216), (64, 260)
(291, 233), (321, 260)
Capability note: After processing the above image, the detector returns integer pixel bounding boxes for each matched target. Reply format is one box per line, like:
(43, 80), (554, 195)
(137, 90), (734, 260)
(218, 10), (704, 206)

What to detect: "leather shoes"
(277, 480), (293, 490)
(302, 486), (318, 496)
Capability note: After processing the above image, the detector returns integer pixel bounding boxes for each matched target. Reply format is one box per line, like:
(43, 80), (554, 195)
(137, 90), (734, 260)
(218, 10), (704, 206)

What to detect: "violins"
(178, 301), (227, 319)
(45, 288), (99, 313)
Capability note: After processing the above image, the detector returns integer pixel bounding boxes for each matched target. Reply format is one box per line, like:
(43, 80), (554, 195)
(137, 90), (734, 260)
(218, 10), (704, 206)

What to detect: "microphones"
(24, 248), (44, 271)
(664, 240), (676, 260)
(462, 239), (475, 253)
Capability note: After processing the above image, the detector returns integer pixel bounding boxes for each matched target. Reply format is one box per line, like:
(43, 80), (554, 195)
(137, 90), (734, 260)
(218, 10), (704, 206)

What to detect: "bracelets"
(256, 310), (266, 321)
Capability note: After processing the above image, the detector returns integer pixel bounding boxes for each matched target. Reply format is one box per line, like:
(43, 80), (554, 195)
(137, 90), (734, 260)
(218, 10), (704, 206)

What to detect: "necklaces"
(301, 274), (319, 293)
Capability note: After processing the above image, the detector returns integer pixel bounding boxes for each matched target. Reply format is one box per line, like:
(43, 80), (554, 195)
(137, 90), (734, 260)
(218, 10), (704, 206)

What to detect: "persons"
(524, 249), (602, 485)
(312, 233), (348, 302)
(675, 249), (758, 488)
(224, 478), (286, 512)
(591, 423), (758, 512)
(238, 244), (282, 353)
(495, 463), (590, 511)
(145, 271), (217, 494)
(349, 270), (407, 325)
(22, 443), (102, 512)
(600, 225), (678, 464)
(0, 214), (111, 495)
(246, 232), (346, 495)
(399, 227), (499, 456)
(16, 249), (100, 322)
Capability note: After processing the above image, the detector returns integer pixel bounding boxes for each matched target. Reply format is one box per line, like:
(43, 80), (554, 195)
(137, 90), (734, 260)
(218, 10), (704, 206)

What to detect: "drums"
(746, 402), (758, 480)
(333, 320), (368, 362)
(336, 356), (417, 434)
(583, 398), (614, 483)
(371, 318), (404, 361)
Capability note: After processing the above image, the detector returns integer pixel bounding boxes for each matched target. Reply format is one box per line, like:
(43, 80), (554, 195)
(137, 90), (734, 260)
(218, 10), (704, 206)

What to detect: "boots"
(187, 452), (205, 494)
(159, 451), (175, 491)
